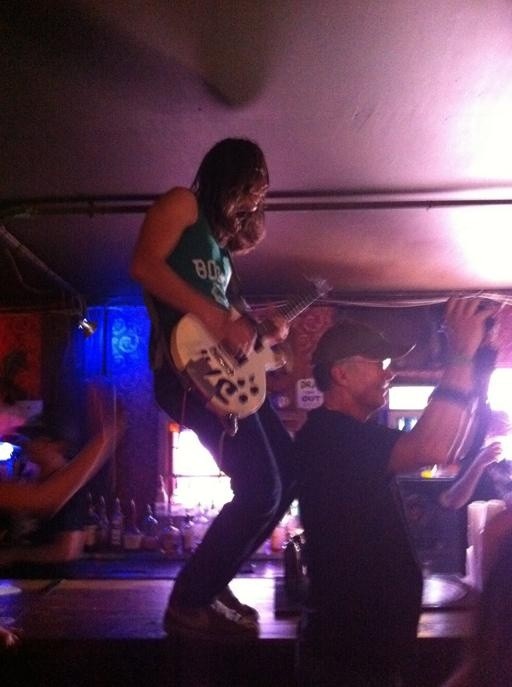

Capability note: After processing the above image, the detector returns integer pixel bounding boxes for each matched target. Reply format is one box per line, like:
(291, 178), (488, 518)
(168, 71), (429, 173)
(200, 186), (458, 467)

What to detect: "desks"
(7, 578), (473, 687)
(0, 578), (56, 687)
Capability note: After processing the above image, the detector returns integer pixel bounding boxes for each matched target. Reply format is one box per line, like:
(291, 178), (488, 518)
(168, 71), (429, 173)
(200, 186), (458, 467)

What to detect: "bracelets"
(424, 383), (473, 415)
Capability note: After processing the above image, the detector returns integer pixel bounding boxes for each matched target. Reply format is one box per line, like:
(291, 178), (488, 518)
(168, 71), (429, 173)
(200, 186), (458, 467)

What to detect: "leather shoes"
(162, 600), (260, 644)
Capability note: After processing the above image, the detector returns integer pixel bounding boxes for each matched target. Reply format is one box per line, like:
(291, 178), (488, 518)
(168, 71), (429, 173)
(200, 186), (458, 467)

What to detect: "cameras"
(437, 305), (497, 374)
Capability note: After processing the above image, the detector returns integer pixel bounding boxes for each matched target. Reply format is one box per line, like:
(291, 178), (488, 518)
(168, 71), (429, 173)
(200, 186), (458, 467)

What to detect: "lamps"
(71, 313), (97, 337)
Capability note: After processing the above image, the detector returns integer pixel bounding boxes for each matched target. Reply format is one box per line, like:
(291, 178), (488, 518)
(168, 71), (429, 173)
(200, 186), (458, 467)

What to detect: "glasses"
(338, 358), (392, 371)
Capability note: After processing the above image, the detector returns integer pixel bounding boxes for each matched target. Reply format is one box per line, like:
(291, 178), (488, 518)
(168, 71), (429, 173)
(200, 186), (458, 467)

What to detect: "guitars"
(165, 270), (335, 438)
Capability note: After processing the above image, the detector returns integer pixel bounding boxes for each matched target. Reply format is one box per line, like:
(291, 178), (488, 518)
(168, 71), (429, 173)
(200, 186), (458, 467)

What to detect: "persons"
(0, 405), (94, 564)
(0, 382), (111, 513)
(434, 410), (511, 579)
(283, 294), (506, 685)
(124, 134), (294, 639)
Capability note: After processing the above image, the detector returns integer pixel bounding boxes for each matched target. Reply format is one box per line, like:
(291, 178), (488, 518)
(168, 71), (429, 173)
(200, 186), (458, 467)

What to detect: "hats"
(309, 320), (415, 365)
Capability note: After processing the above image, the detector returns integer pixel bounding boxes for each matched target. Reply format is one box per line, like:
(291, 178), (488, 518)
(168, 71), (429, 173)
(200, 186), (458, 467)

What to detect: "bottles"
(85, 482), (304, 554)
